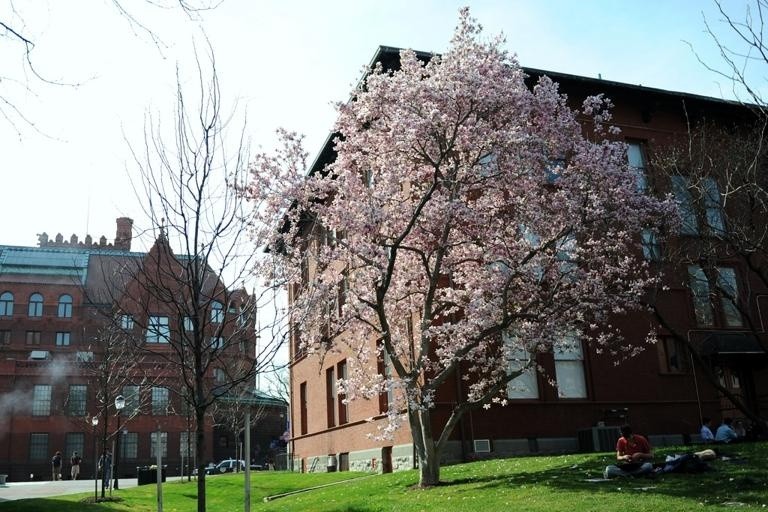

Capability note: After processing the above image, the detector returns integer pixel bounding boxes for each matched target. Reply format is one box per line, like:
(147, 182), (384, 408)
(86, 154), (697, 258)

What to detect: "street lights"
(279, 406), (284, 437)
(92, 416), (99, 502)
(113, 394), (126, 488)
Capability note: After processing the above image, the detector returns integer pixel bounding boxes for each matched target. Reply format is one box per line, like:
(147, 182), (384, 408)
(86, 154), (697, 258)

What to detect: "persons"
(603, 424), (655, 481)
(715, 417), (738, 444)
(97, 446), (113, 489)
(701, 417), (714, 442)
(69, 451), (83, 479)
(51, 451), (62, 481)
(734, 419), (746, 441)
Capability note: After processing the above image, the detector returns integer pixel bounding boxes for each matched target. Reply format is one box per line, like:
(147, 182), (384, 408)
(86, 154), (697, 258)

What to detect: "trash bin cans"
(136, 465), (168, 486)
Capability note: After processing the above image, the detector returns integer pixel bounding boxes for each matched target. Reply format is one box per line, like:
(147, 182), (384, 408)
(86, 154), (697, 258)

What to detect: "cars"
(192, 458), (263, 476)
